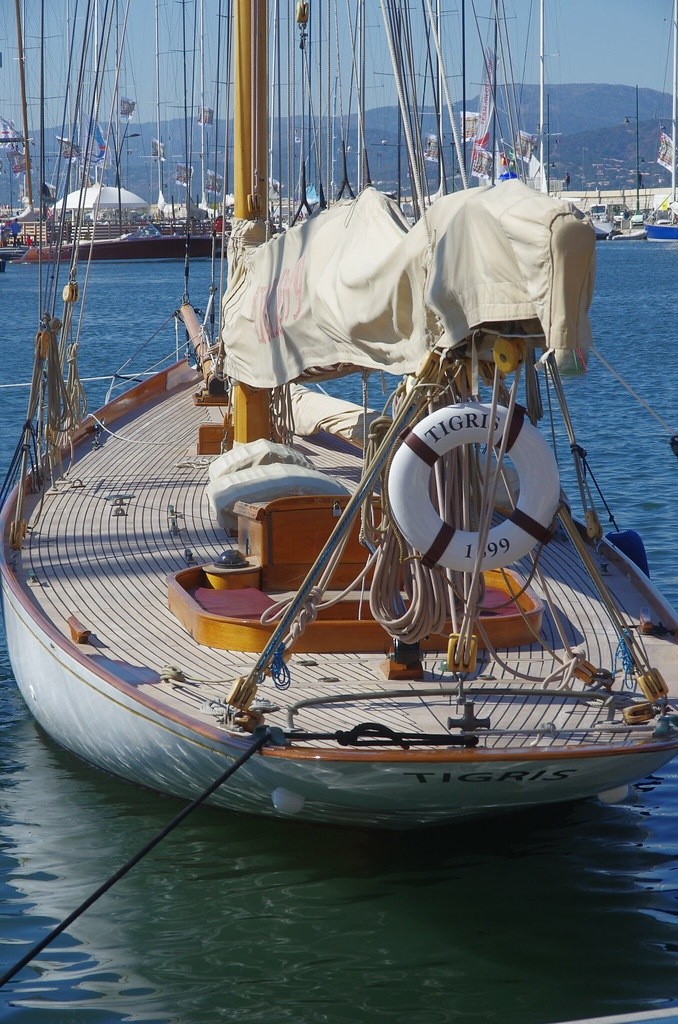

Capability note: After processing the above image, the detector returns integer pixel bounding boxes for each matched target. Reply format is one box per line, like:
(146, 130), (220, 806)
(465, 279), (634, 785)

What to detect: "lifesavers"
(388, 402), (560, 572)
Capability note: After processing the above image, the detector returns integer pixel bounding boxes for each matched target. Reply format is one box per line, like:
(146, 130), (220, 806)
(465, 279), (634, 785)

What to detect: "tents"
(51, 182), (150, 227)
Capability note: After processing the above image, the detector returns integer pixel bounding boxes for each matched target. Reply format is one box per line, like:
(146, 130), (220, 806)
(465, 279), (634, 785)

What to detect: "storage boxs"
(231, 494), (407, 593)
(196, 423), (235, 455)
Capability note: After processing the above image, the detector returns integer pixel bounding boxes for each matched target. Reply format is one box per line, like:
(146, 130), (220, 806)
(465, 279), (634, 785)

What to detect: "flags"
(151, 137), (167, 162)
(198, 105), (214, 127)
(176, 162), (194, 187)
(80, 113), (112, 171)
(268, 178), (283, 201)
(423, 134), (440, 163)
(295, 137), (301, 143)
(656, 132), (673, 172)
(0, 120), (32, 173)
(56, 136), (81, 166)
(459, 42), (537, 180)
(204, 169), (224, 197)
(120, 96), (137, 120)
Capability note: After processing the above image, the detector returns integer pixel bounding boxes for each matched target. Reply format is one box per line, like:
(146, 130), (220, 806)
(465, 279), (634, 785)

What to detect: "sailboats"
(0, 0), (678, 264)
(0, 1), (678, 829)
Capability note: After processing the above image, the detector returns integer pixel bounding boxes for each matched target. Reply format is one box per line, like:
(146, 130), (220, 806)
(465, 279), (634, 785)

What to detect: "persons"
(25, 234), (32, 247)
(10, 218), (22, 248)
(623, 209), (629, 220)
(566, 173), (570, 191)
(637, 171), (642, 188)
(658, 177), (662, 187)
